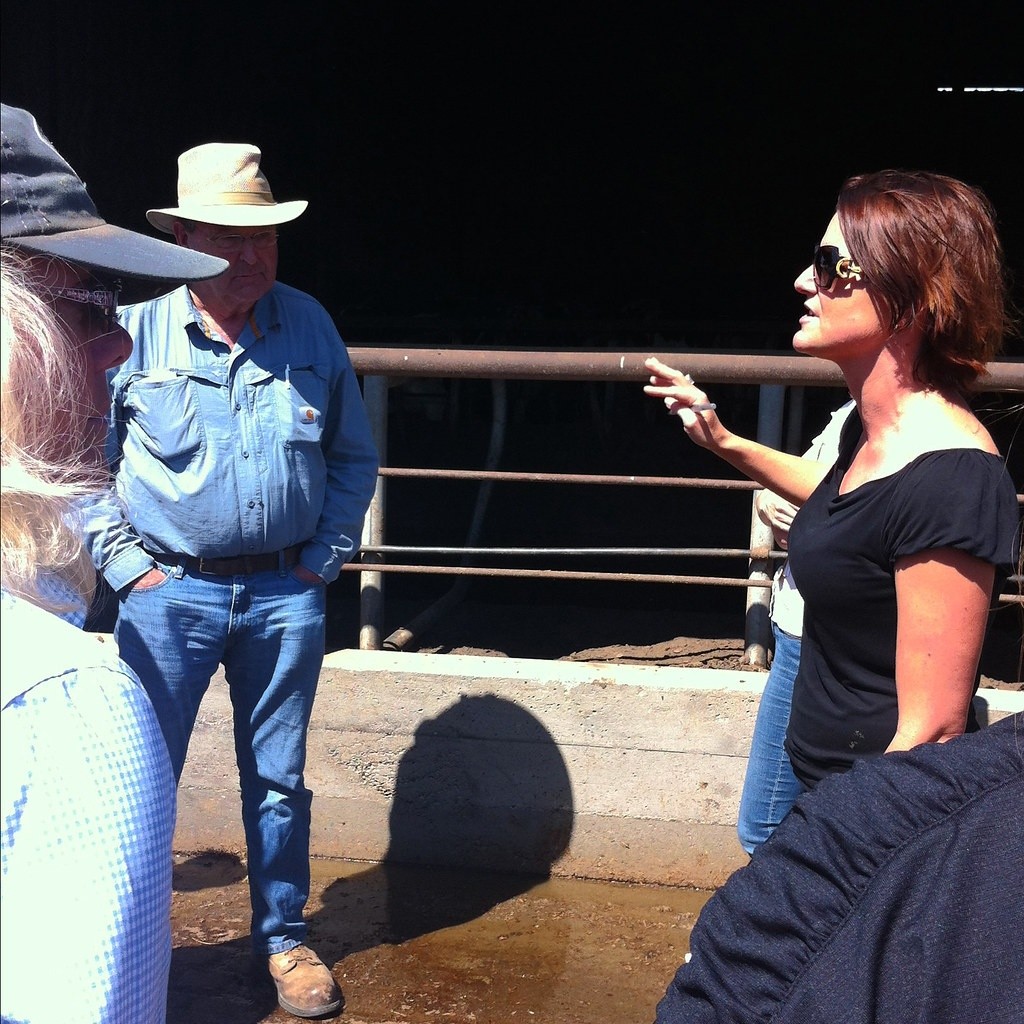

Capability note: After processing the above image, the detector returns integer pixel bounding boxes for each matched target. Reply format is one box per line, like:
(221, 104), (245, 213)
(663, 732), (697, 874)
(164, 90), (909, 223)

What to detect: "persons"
(643, 168), (1023, 862)
(1, 103), (230, 1024)
(652, 712), (1024, 1024)
(72, 143), (378, 1019)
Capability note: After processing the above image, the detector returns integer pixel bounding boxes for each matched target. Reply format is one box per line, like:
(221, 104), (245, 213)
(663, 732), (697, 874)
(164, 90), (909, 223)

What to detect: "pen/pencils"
(667, 403), (717, 416)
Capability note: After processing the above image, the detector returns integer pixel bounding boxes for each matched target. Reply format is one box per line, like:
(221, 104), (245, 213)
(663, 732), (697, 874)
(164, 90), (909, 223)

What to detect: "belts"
(152, 537), (311, 577)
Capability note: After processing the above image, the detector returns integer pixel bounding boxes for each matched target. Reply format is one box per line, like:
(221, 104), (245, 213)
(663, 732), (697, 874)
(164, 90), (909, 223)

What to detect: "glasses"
(192, 227), (280, 248)
(810, 244), (865, 292)
(47, 277), (122, 334)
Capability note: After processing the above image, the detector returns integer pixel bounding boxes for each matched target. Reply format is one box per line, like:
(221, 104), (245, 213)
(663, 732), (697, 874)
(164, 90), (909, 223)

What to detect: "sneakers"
(250, 940), (342, 1017)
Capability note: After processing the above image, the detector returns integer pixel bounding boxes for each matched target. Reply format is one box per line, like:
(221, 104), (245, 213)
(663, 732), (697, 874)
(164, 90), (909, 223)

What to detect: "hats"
(0, 102), (230, 281)
(146, 141), (309, 235)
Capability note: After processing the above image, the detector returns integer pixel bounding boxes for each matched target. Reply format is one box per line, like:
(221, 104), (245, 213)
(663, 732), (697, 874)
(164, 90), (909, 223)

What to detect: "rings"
(685, 374), (694, 385)
